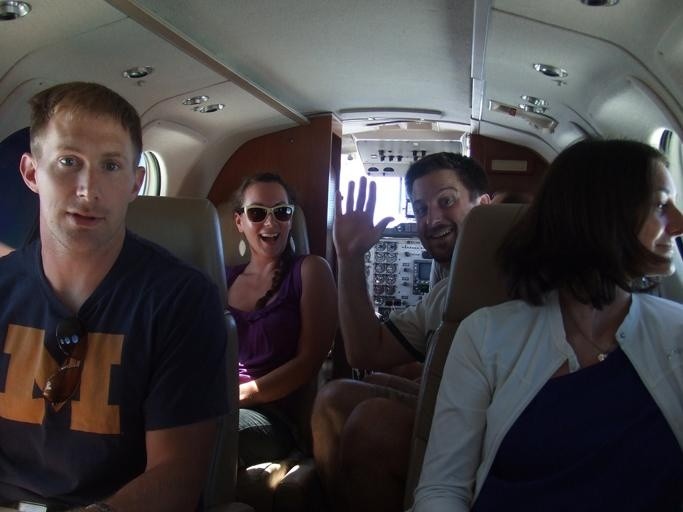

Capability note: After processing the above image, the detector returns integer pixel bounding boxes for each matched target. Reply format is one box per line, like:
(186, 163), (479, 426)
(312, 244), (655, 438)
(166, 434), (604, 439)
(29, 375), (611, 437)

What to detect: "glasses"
(44, 315), (88, 401)
(239, 205), (295, 224)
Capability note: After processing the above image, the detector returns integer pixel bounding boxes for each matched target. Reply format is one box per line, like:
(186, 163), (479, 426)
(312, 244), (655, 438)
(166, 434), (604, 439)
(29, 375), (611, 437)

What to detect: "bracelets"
(91, 502), (114, 512)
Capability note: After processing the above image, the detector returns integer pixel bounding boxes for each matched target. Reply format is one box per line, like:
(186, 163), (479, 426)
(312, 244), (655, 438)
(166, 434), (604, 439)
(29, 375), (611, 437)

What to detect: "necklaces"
(563, 306), (620, 363)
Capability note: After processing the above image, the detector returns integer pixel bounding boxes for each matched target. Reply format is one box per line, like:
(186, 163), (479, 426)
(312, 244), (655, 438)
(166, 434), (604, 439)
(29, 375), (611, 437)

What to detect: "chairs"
(124, 189), (310, 512)
(407, 202), (536, 511)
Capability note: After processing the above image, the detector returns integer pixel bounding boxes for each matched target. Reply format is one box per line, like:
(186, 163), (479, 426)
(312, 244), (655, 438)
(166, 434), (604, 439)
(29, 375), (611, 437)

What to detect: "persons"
(0, 81), (228, 512)
(218, 172), (338, 471)
(311, 151), (493, 511)
(414, 137), (683, 511)
